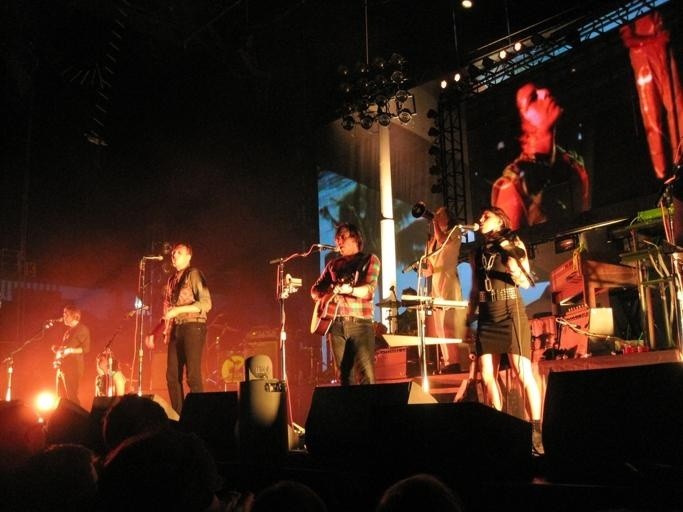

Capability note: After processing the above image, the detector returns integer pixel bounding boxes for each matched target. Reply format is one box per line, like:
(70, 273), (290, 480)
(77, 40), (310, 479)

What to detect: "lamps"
(338, 33), (547, 194)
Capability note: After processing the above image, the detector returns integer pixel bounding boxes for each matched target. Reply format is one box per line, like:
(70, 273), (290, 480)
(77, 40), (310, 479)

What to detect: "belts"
(335, 314), (372, 324)
(172, 318), (207, 327)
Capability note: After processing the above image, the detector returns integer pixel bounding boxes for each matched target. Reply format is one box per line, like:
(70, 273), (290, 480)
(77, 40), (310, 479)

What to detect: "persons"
(453, 207), (544, 447)
(616, 7), (682, 185)
(309, 224), (382, 385)
(53, 305), (91, 407)
(160, 241), (212, 413)
(93, 350), (125, 396)
(1, 395), (457, 511)
(416, 205), (467, 374)
(480, 77), (591, 231)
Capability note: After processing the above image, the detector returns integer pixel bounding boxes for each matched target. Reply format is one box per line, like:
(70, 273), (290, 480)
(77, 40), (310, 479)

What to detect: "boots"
(532, 419), (544, 456)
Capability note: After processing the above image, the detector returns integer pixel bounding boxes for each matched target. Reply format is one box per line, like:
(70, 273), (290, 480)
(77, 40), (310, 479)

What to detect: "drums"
(221, 355), (245, 383)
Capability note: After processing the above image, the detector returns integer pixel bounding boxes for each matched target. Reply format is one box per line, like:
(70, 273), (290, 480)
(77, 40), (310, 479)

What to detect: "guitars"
(311, 254), (371, 336)
(145, 301), (174, 349)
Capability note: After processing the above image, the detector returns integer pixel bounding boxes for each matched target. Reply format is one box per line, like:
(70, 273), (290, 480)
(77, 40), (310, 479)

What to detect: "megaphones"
(411, 200), (436, 222)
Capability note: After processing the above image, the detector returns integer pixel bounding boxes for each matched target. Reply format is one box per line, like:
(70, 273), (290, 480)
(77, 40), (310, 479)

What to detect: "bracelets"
(348, 287), (354, 296)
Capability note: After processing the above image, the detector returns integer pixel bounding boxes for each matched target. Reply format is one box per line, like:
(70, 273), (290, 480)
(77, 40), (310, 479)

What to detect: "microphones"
(47, 316), (65, 325)
(321, 241), (342, 257)
(140, 252), (166, 264)
(458, 221), (482, 234)
(131, 304), (151, 315)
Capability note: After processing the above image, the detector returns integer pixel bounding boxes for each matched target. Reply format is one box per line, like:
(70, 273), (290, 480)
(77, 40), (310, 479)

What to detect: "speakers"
(91, 393), (155, 449)
(178, 390), (239, 433)
(407, 401), (534, 488)
(303, 379), (414, 451)
(539, 361), (683, 489)
